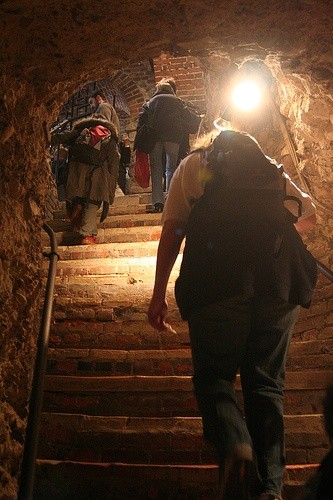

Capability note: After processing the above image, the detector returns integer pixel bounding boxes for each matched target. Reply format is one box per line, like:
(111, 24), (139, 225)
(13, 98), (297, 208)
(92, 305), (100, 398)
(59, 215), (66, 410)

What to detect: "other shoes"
(119, 184), (128, 195)
(154, 202), (164, 212)
(258, 493), (282, 500)
(72, 234), (82, 244)
(80, 235), (100, 245)
(221, 443), (254, 500)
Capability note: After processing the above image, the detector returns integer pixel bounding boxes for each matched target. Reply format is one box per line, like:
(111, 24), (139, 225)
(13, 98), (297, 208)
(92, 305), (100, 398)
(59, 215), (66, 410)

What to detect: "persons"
(48, 112), (124, 246)
(87, 90), (130, 197)
(305, 384), (333, 500)
(132, 76), (206, 213)
(147, 129), (320, 500)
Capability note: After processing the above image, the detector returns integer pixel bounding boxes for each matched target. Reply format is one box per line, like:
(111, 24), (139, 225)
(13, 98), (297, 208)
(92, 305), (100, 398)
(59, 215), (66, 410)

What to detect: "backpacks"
(182, 145), (302, 222)
(69, 123), (116, 168)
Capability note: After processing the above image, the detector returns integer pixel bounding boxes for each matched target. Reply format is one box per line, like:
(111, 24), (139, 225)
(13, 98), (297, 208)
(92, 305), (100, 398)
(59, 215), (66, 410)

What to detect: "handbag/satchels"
(135, 150), (150, 188)
(185, 104), (200, 134)
(133, 106), (156, 152)
